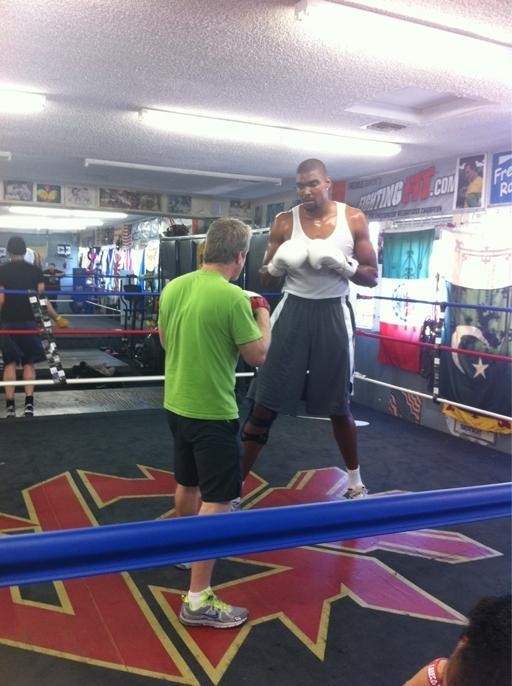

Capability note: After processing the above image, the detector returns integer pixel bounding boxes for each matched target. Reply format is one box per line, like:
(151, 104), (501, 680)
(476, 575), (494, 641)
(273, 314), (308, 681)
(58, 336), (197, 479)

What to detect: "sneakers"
(24, 404), (33, 416)
(230, 498), (241, 513)
(344, 487), (367, 500)
(7, 406), (16, 417)
(180, 595), (248, 628)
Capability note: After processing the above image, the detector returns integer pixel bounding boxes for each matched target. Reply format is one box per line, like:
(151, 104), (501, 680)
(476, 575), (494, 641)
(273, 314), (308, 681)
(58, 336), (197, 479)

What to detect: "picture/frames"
(453, 152), (487, 212)
(34, 181), (64, 204)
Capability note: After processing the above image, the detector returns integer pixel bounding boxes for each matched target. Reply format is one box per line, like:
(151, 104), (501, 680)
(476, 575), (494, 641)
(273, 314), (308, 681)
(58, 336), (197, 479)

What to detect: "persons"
(459, 160), (483, 208)
(230, 159), (378, 512)
(402, 596), (512, 686)
(0, 237), (69, 419)
(157, 217), (273, 630)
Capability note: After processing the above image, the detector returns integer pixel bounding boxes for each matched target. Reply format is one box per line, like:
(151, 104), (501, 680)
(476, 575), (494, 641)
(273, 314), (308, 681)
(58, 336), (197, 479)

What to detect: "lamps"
(290, 0), (510, 75)
(136, 108), (401, 160)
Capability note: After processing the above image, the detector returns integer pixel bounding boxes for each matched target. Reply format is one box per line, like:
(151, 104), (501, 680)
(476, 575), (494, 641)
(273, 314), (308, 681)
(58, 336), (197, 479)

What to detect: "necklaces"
(427, 657), (445, 686)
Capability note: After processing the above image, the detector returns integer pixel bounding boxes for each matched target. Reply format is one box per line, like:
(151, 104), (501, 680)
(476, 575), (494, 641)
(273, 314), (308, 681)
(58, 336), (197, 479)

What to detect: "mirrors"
(1, 202), (220, 338)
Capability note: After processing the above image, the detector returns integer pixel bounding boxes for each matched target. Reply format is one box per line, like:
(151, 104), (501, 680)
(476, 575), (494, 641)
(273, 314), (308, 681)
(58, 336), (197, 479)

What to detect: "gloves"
(241, 290), (270, 313)
(267, 240), (309, 278)
(308, 238), (359, 278)
